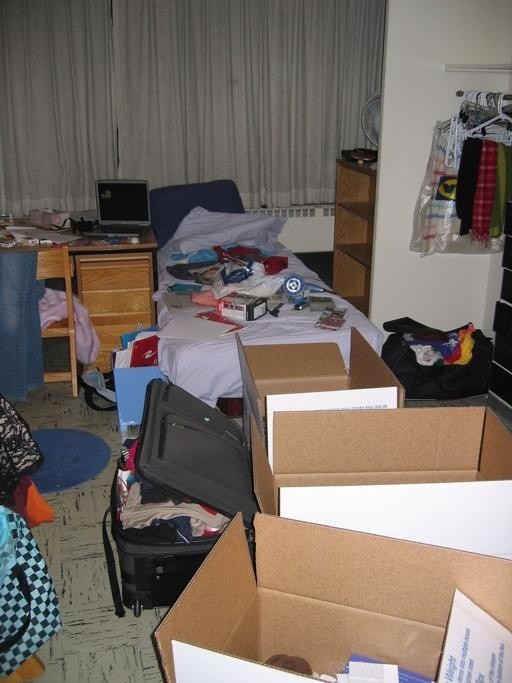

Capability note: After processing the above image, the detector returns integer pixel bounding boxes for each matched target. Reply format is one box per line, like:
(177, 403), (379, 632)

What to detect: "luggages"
(109, 378), (258, 618)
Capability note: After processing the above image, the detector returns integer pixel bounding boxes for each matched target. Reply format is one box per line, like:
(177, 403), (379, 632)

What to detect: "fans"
(361, 93), (380, 169)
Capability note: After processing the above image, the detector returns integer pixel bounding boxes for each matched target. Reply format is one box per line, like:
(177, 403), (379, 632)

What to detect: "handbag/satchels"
(0, 392), (63, 678)
(381, 317), (493, 400)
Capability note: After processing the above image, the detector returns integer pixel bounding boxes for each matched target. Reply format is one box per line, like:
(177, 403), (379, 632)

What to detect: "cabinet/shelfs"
(3, 223), (158, 374)
(331, 159), (376, 319)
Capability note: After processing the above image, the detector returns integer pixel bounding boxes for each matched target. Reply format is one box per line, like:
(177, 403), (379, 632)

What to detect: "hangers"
(441, 90), (512, 144)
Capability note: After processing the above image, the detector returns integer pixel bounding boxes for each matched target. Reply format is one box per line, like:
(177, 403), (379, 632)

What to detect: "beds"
(149, 180), (382, 417)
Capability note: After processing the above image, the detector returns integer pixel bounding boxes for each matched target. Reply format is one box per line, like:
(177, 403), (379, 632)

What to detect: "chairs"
(0, 246), (78, 398)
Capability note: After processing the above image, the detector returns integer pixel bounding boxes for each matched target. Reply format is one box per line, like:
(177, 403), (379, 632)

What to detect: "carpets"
(24, 429), (111, 494)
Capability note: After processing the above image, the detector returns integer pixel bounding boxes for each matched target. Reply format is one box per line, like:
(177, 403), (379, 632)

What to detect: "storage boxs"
(150, 510), (512, 683)
(111, 351), (168, 444)
(233, 327), (406, 466)
(246, 408), (512, 559)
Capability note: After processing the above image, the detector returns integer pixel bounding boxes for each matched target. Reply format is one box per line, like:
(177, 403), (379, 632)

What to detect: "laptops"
(82, 179), (151, 237)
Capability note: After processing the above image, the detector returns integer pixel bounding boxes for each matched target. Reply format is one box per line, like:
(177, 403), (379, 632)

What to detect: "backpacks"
(79, 367), (117, 411)
